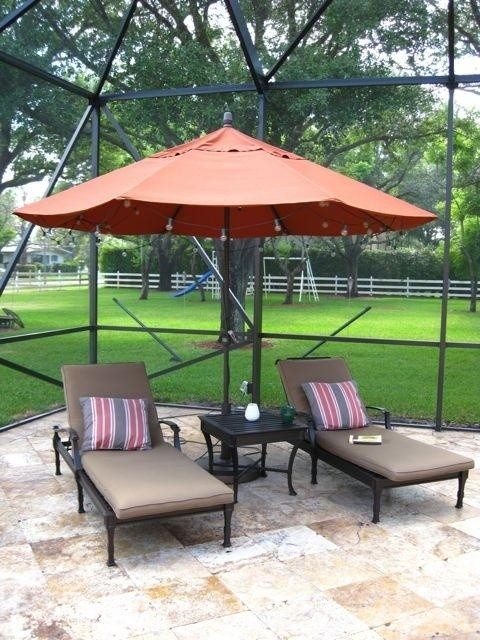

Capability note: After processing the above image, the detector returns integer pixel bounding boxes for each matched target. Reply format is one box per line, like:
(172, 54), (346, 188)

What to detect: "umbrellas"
(11, 112), (438, 459)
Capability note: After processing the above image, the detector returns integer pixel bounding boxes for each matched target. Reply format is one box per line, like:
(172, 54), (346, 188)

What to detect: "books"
(349, 435), (382, 444)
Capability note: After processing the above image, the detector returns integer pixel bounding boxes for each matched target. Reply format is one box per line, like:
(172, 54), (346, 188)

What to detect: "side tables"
(198, 411), (312, 504)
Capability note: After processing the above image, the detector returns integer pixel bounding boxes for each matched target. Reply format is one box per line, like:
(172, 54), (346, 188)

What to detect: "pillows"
(79, 397), (153, 452)
(300, 380), (372, 431)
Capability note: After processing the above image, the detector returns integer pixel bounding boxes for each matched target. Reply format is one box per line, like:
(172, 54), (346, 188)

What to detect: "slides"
(170, 270), (213, 298)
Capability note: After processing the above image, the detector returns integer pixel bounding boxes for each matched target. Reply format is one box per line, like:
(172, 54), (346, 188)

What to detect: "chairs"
(54, 363), (236, 566)
(275, 356), (474, 524)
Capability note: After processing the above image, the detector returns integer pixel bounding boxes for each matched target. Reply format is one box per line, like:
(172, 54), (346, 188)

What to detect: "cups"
(280, 405), (296, 424)
